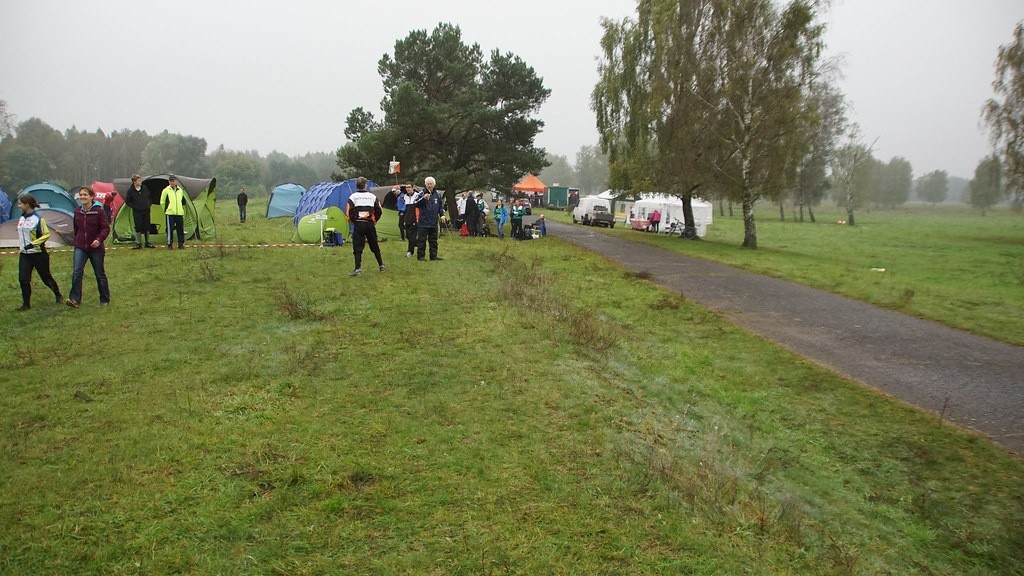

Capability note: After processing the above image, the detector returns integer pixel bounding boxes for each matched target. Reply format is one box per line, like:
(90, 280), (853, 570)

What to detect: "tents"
(0, 173), (219, 249)
(626, 194), (713, 238)
(265, 178), (377, 245)
(513, 174), (547, 193)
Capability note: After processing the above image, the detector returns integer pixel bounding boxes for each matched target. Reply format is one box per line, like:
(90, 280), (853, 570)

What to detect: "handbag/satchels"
(334, 232), (343, 246)
(461, 224), (469, 235)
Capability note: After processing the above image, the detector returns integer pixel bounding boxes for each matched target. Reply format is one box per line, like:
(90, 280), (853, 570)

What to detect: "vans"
(491, 188), (503, 202)
(572, 197), (611, 224)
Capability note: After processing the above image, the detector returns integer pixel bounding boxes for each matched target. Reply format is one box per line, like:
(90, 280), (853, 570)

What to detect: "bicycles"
(667, 217), (698, 240)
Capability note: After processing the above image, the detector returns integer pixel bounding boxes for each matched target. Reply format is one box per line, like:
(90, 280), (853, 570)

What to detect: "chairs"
(530, 223), (542, 237)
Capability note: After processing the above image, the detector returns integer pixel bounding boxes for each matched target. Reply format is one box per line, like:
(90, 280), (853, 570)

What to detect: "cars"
(514, 198), (532, 215)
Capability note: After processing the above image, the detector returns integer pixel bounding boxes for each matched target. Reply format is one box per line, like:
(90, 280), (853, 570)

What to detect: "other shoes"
(100, 302), (108, 308)
(380, 264), (386, 272)
(132, 243), (141, 250)
(145, 242), (157, 248)
(179, 242), (184, 249)
(420, 256), (427, 262)
(66, 299), (79, 307)
(350, 268), (361, 277)
(431, 257), (444, 261)
(56, 295), (63, 304)
(167, 244), (173, 250)
(405, 251), (412, 257)
(16, 305), (30, 311)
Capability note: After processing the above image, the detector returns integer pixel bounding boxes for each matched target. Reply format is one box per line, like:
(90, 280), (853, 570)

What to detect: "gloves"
(24, 243), (33, 250)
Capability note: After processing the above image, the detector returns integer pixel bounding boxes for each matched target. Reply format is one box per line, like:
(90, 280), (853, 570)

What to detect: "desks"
(630, 219), (652, 231)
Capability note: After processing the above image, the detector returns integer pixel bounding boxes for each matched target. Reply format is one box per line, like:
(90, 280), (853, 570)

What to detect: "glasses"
(405, 187), (412, 189)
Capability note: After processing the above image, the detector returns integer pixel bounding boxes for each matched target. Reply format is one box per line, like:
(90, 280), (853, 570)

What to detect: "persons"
(413, 176), (446, 261)
(455, 190), (547, 239)
(392, 185), (406, 241)
(66, 186), (111, 308)
(344, 177), (386, 277)
(237, 187), (248, 222)
(125, 173), (155, 251)
(159, 175), (187, 250)
(403, 181), (419, 258)
(649, 208), (661, 235)
(16, 195), (63, 312)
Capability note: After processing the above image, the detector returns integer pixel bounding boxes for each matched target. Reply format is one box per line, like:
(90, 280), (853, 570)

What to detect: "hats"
(169, 175), (176, 180)
(357, 177), (370, 183)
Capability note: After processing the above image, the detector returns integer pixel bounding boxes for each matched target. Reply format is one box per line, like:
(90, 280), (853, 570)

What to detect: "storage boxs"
(329, 232), (343, 245)
